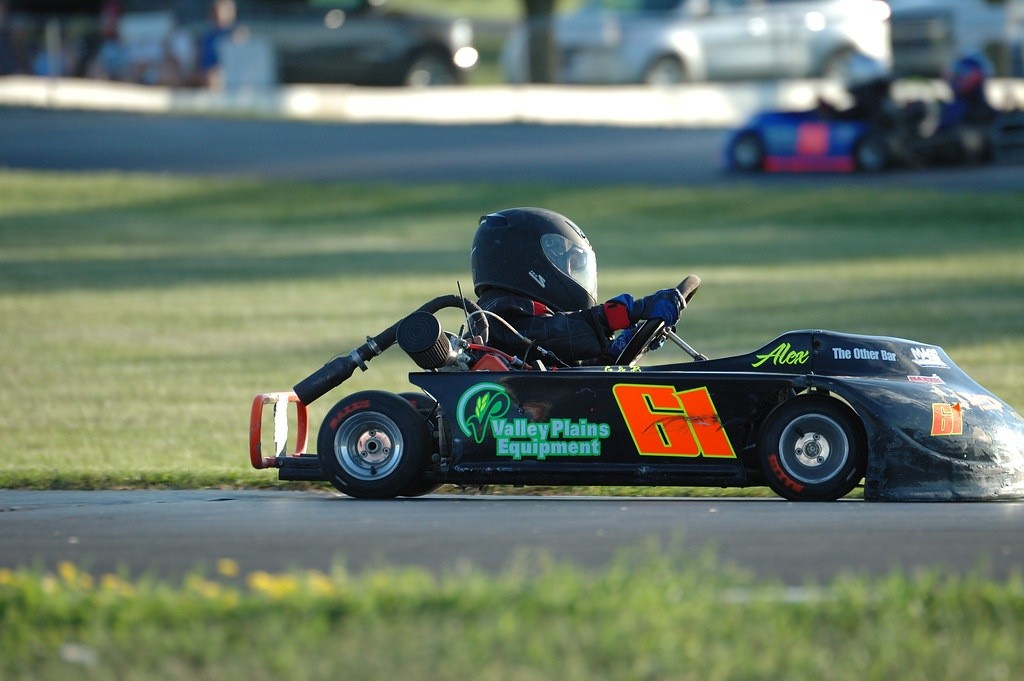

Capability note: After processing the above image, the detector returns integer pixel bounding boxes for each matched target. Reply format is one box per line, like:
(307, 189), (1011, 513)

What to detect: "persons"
(470, 207), (687, 365)
(941, 53), (994, 125)
(0, 1), (279, 101)
(805, 26), (889, 111)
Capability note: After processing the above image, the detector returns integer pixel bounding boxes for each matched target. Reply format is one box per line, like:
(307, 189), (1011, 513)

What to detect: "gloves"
(611, 322), (676, 357)
(604, 288), (687, 327)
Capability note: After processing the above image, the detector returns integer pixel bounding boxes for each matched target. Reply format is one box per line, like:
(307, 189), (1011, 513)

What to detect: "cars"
(239, 0), (473, 86)
(500, 1), (1024, 111)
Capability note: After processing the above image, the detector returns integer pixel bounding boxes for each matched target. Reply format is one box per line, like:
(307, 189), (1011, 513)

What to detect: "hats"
(471, 207), (597, 312)
